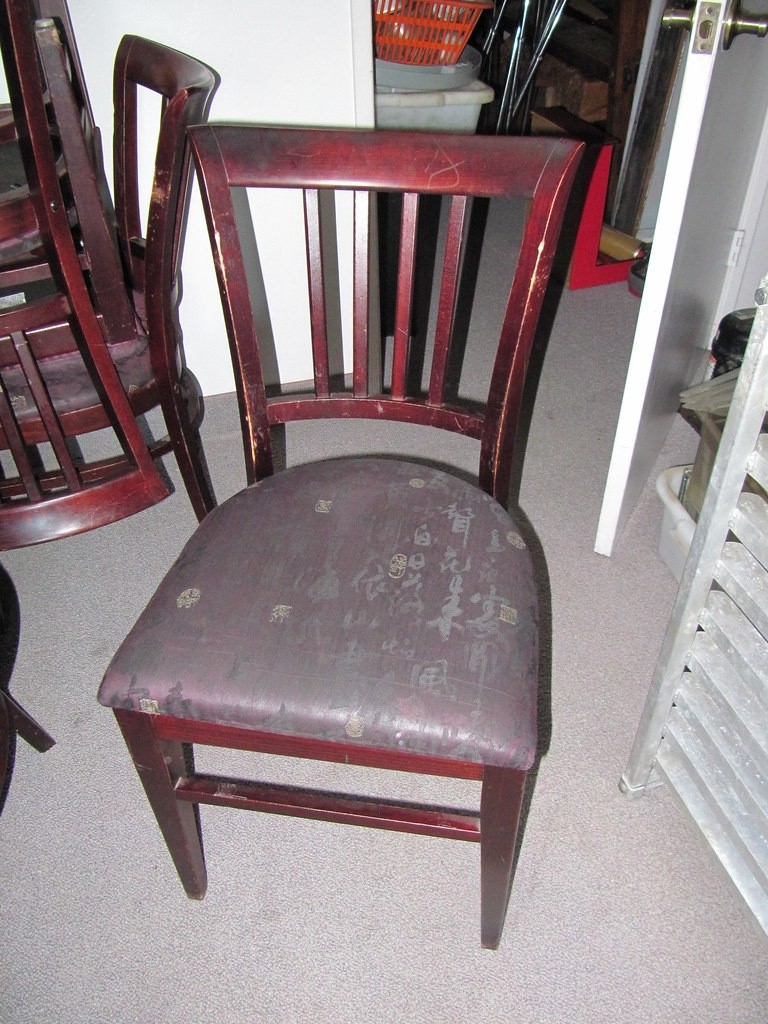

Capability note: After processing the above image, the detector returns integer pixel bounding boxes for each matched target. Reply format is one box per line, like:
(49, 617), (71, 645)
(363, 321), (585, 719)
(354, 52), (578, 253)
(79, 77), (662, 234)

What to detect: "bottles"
(599, 223), (646, 261)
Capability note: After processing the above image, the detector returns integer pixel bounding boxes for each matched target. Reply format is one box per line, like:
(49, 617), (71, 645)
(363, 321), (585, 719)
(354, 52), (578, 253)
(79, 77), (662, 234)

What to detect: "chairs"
(1, 0), (221, 557)
(96, 121), (587, 951)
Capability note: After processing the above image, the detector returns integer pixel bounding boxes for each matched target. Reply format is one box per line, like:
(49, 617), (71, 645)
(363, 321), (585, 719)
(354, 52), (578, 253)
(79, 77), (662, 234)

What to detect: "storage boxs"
(655, 462), (699, 587)
(677, 404), (726, 524)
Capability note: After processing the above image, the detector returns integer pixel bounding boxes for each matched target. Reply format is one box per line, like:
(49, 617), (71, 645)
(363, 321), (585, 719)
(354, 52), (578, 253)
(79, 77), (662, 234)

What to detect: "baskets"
(372, 0), (495, 66)
